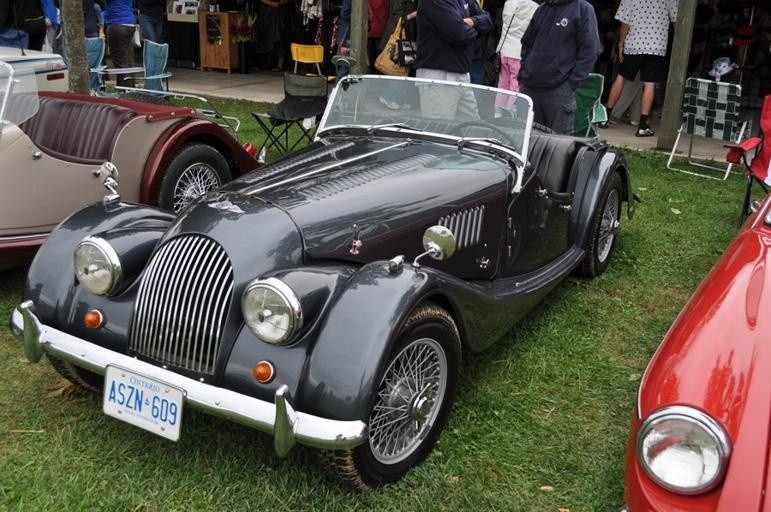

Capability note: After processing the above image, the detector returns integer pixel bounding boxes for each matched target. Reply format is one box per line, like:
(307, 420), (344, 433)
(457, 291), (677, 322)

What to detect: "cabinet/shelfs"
(198, 10), (271, 74)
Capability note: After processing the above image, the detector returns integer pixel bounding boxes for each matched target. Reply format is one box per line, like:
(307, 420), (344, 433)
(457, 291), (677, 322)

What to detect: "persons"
(1, 0), (166, 87)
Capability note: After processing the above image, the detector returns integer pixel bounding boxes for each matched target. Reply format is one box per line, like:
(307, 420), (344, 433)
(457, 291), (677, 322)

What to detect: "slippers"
(270, 66), (286, 73)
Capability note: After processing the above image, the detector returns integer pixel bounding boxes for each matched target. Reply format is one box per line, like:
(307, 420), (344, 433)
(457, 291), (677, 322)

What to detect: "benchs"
(2, 95), (137, 166)
(382, 114), (576, 198)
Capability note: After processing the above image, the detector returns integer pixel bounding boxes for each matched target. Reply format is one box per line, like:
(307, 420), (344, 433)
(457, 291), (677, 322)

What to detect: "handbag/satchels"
(397, 16), (420, 67)
(267, 1), (298, 40)
(130, 26), (142, 50)
(373, 16), (411, 79)
(490, 51), (502, 73)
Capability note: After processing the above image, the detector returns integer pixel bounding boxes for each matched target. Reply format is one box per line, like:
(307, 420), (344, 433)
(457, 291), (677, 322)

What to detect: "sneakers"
(598, 117), (655, 137)
(378, 96), (413, 112)
(492, 107), (519, 121)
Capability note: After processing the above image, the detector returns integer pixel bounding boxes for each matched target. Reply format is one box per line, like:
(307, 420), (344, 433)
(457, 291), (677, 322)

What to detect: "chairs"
(569, 73), (608, 142)
(720, 94), (771, 222)
(667, 75), (755, 183)
(75, 23), (338, 165)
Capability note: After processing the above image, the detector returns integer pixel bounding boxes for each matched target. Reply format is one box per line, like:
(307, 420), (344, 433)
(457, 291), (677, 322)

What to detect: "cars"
(622, 191), (770, 512)
(11, 64), (636, 486)
(1, 57), (259, 276)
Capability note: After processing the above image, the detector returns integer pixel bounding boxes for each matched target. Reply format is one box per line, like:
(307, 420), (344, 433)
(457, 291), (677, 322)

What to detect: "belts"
(119, 22), (141, 28)
